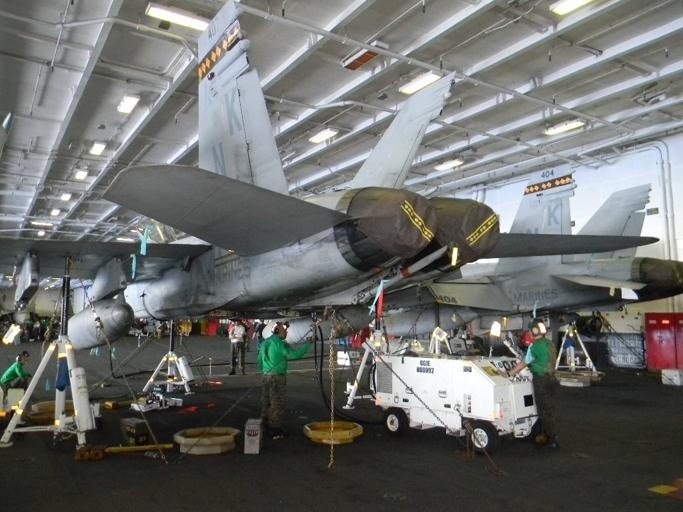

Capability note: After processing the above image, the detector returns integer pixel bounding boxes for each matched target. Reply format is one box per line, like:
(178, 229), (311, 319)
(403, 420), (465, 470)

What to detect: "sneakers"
(228, 368), (236, 376)
(239, 368), (245, 376)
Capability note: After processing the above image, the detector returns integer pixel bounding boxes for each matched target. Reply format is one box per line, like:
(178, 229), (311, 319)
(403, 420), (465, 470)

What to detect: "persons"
(496, 319), (557, 443)
(227, 319), (267, 375)
(0, 351), (40, 411)
(257, 322), (313, 440)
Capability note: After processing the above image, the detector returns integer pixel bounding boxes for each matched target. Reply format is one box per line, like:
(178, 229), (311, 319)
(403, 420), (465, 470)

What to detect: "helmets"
(530, 322), (548, 338)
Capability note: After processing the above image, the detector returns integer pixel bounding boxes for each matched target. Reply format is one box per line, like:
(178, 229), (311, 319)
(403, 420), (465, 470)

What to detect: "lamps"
(435, 156), (467, 173)
(49, 94), (139, 216)
(307, 127), (337, 145)
(143, 3), (210, 32)
(396, 70), (443, 97)
(541, 118), (586, 137)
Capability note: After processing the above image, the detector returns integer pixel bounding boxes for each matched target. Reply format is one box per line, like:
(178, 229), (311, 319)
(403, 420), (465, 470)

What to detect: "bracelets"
(507, 372), (510, 377)
